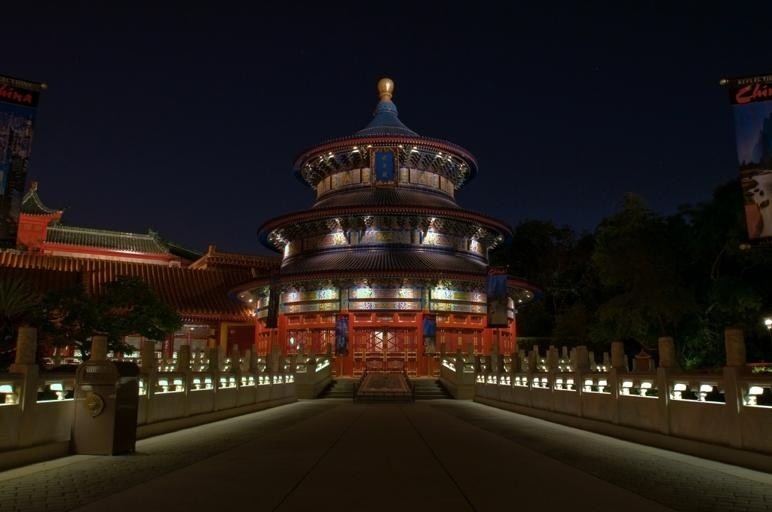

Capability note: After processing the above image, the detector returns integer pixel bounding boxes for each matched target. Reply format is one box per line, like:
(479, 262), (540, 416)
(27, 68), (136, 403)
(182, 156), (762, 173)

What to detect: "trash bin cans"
(68, 359), (140, 457)
